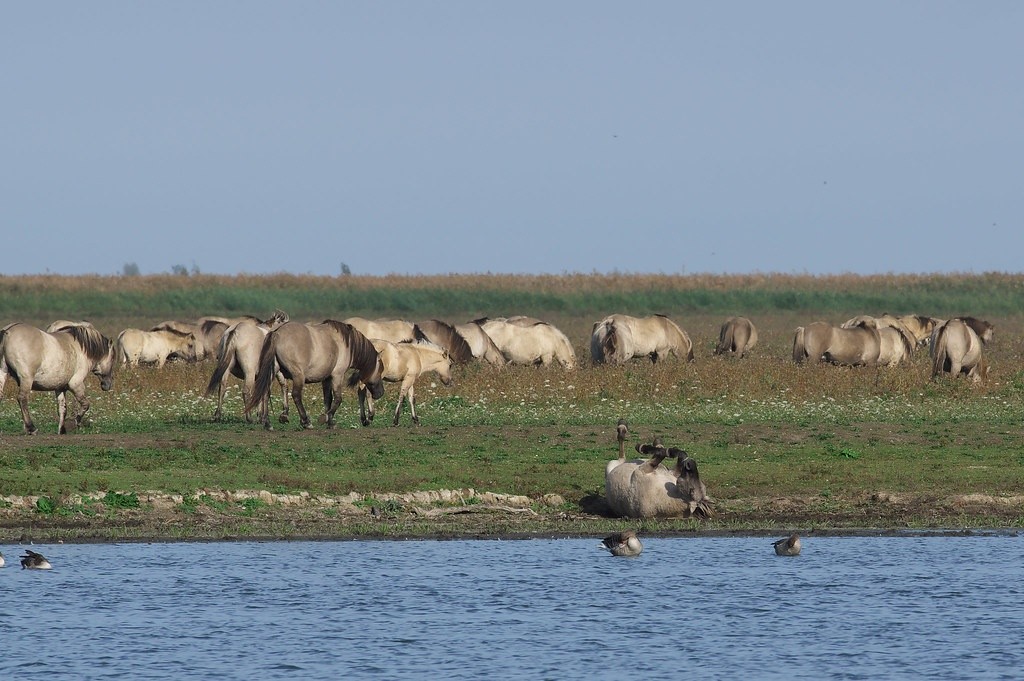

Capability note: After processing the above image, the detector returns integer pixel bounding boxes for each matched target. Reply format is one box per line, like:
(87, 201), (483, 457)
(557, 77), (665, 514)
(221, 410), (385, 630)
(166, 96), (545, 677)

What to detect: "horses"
(604, 419), (716, 520)
(716, 316), (758, 359)
(792, 313), (995, 384)
(0, 308), (577, 438)
(591, 312), (693, 367)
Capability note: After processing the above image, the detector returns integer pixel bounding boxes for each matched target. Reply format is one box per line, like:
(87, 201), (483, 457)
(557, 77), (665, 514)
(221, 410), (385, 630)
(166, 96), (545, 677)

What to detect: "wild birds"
(602, 528), (642, 556)
(18, 549), (53, 571)
(771, 532), (801, 556)
